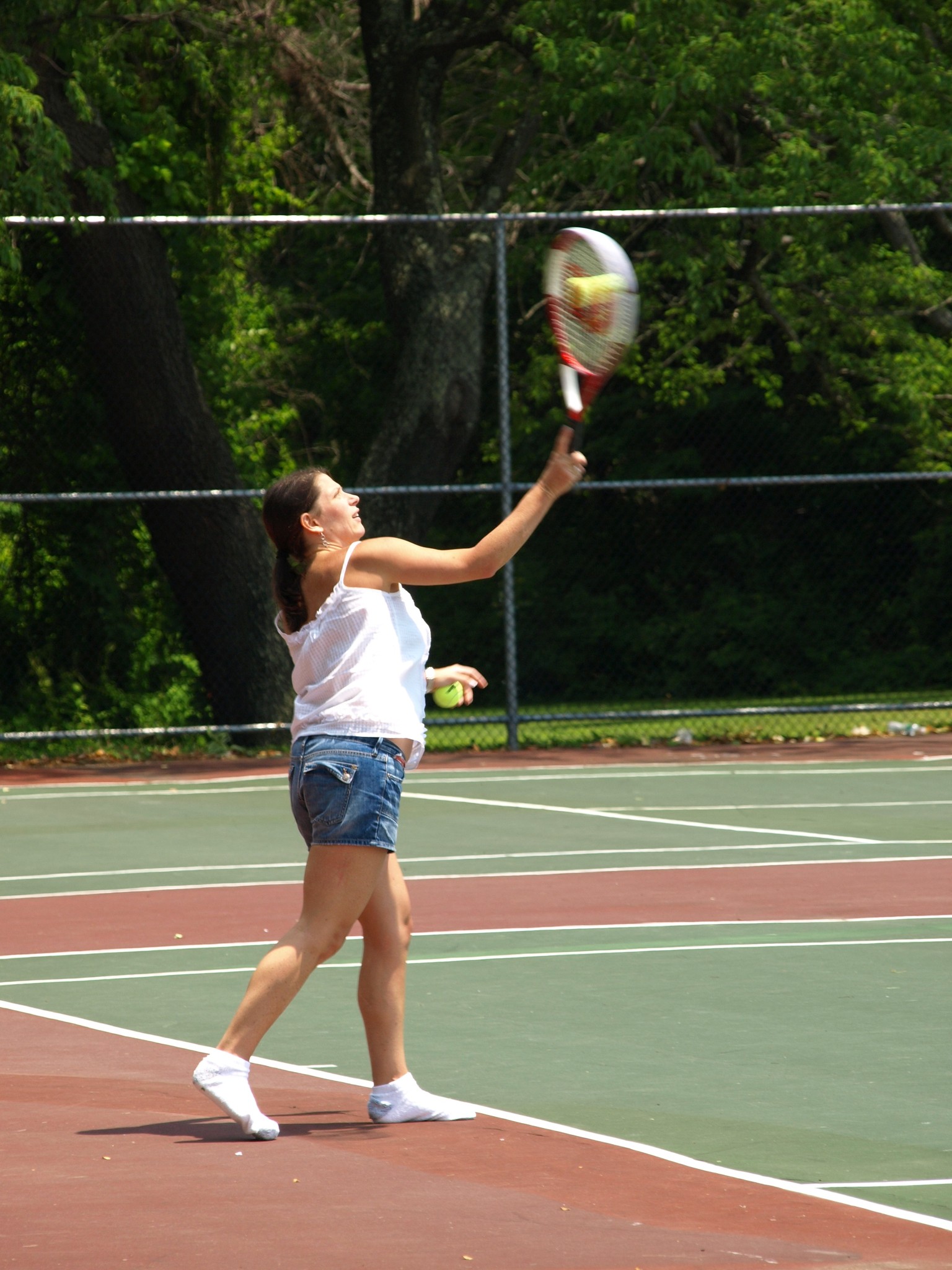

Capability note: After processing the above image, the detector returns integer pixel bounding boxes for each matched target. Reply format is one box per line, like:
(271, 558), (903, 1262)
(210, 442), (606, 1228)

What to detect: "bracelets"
(425, 666), (436, 694)
(535, 479), (559, 503)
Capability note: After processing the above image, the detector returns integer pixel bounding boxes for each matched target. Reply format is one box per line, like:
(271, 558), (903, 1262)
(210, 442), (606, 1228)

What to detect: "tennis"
(563, 274), (619, 312)
(435, 682), (466, 711)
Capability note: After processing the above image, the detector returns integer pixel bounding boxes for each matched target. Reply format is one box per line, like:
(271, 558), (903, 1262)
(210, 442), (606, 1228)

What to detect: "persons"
(193, 426), (586, 1137)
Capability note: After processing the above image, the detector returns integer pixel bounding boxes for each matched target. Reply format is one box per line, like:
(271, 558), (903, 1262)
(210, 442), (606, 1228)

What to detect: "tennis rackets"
(544, 224), (637, 467)
